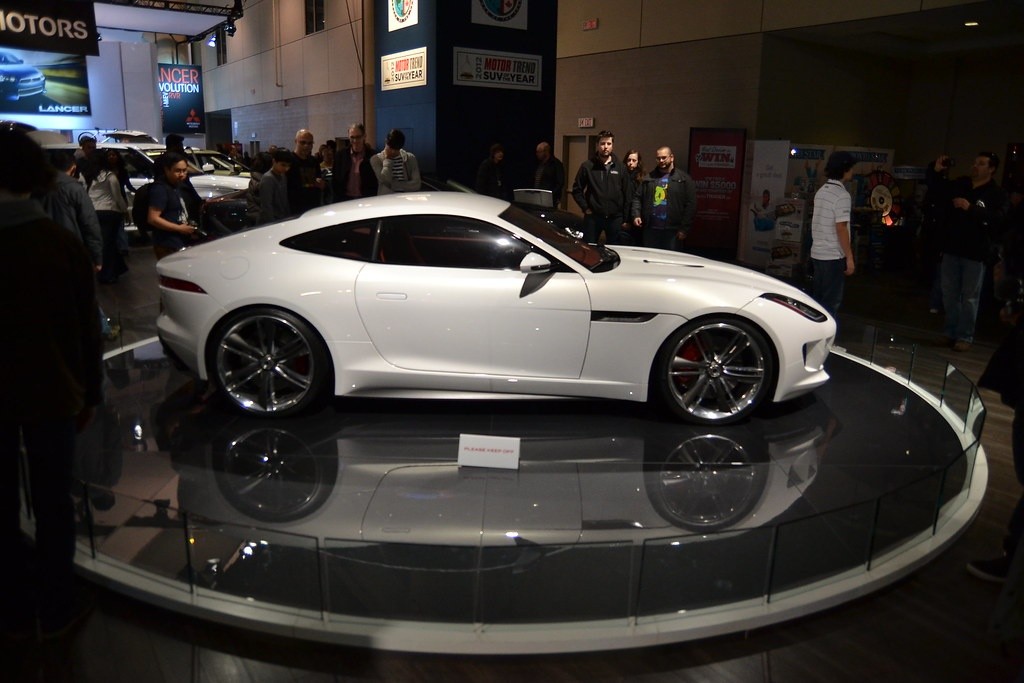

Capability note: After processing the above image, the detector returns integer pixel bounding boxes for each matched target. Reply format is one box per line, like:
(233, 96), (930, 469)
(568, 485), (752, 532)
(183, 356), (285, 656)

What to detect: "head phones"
(78, 132), (97, 147)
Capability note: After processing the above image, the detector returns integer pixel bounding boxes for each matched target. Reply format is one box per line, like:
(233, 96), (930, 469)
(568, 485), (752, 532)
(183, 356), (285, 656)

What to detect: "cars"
(0, 53), (48, 102)
(46, 129), (252, 231)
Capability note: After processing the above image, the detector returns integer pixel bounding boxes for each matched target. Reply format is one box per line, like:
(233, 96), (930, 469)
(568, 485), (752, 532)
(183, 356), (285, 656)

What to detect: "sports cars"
(151, 192), (837, 417)
(152, 382), (840, 553)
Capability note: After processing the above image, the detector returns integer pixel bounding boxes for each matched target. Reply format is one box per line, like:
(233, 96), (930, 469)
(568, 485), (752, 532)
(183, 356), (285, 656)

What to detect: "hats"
(166, 134), (184, 149)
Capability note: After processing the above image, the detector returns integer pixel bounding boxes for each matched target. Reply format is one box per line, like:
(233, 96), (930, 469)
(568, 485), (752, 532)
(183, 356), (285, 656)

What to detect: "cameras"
(188, 221), (207, 240)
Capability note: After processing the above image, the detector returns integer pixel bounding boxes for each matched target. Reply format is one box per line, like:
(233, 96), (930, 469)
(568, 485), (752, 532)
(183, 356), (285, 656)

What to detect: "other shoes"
(965, 557), (1008, 583)
(953, 340), (972, 352)
(933, 335), (955, 347)
(2, 622), (36, 641)
(930, 307), (939, 314)
(38, 611), (90, 640)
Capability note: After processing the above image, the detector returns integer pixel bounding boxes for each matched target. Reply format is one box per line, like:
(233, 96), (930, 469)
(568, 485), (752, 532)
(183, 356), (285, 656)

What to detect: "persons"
(185, 108), (201, 123)
(215, 129), (338, 227)
(927, 150), (1024, 353)
(531, 142), (564, 209)
(810, 150), (855, 337)
(0, 126), (130, 611)
(332, 123), (377, 202)
(371, 128), (420, 195)
(967, 314), (1024, 583)
(478, 143), (506, 199)
(74, 351), (130, 512)
(751, 189), (776, 233)
(146, 132), (202, 261)
(623, 147), (696, 252)
(572, 129), (634, 246)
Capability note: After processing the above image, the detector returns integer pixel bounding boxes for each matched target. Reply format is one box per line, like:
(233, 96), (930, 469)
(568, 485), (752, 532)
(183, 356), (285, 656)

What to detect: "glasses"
(657, 156), (671, 163)
(350, 134), (364, 139)
(298, 139), (313, 147)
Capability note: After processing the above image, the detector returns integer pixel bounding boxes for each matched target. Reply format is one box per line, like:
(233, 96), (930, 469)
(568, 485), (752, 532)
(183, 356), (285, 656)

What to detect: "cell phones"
(942, 158), (954, 167)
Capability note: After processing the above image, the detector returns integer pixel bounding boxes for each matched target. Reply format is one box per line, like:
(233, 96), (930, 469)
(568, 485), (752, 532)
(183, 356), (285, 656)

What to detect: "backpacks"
(132, 183), (169, 242)
